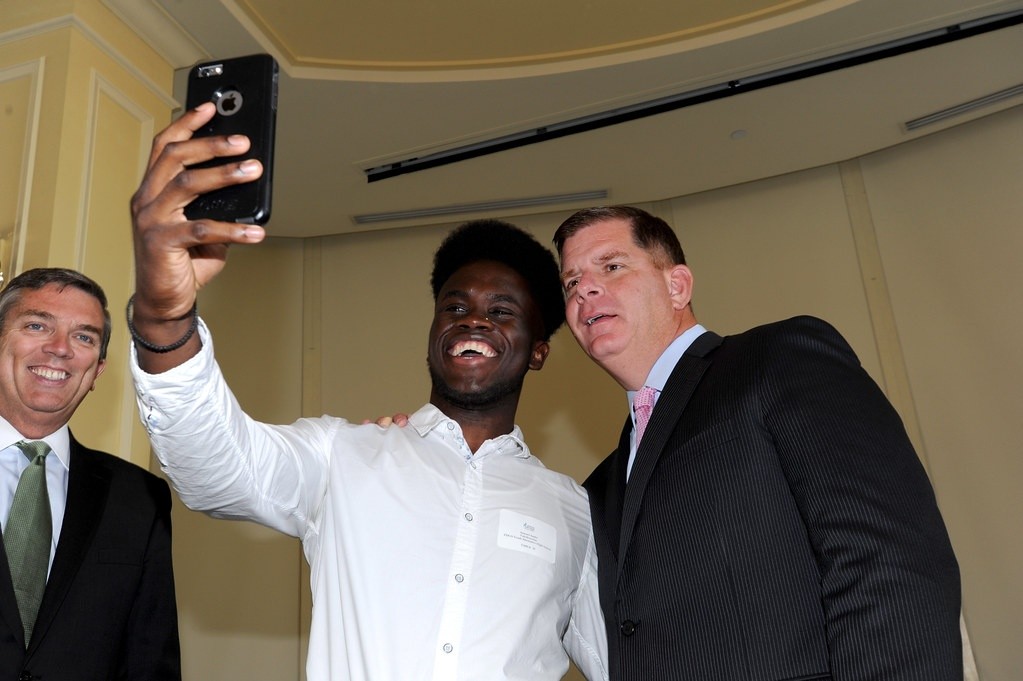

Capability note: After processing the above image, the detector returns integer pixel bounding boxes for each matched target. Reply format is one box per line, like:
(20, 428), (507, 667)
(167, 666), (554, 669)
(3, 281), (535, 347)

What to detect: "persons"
(551, 205), (968, 681)
(0, 266), (186, 681)
(120, 98), (610, 681)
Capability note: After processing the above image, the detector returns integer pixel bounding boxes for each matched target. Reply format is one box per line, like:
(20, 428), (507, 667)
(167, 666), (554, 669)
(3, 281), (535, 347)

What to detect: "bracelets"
(126, 290), (197, 353)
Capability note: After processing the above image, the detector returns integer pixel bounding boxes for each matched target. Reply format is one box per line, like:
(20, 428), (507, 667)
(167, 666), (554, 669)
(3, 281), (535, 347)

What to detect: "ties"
(2, 440), (53, 649)
(634, 386), (657, 451)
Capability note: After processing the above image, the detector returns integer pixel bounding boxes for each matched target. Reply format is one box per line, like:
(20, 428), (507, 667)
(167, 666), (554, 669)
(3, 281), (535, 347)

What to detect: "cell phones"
(185, 52), (279, 227)
